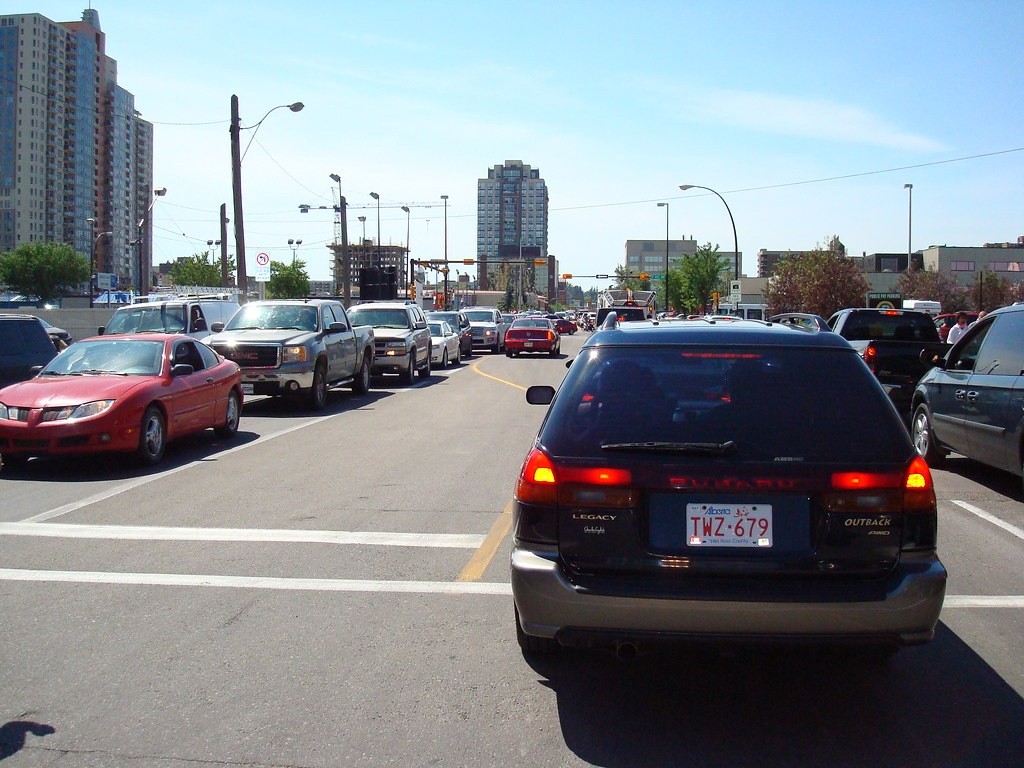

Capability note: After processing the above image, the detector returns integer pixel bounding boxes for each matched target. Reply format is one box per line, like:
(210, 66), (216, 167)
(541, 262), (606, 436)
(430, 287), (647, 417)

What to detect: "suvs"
(343, 299), (433, 385)
(511, 311), (949, 654)
(0, 312), (60, 390)
(92, 290), (141, 304)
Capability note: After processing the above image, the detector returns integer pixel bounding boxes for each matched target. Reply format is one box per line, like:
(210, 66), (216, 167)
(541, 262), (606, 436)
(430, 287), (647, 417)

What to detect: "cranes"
(329, 186), (446, 244)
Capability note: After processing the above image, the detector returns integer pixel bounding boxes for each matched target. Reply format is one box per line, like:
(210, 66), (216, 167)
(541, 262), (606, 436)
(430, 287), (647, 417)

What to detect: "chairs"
(896, 325), (914, 338)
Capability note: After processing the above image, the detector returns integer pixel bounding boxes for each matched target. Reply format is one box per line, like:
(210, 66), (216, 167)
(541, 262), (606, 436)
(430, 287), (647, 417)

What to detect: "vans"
(97, 298), (240, 350)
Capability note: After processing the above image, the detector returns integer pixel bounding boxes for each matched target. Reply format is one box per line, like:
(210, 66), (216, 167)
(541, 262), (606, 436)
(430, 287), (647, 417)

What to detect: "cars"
(423, 319), (462, 369)
(905, 300), (1024, 482)
(37, 317), (72, 350)
(0, 334), (245, 468)
(0, 291), (43, 302)
(423, 303), (1023, 355)
(504, 317), (561, 358)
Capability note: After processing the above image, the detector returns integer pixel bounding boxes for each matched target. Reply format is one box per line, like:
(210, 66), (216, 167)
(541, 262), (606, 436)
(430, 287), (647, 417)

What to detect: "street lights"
(441, 195), (449, 311)
(85, 219), (114, 308)
(287, 238), (302, 265)
(401, 205), (410, 300)
(369, 192), (381, 298)
(679, 184), (739, 316)
(657, 202), (669, 310)
(228, 94), (305, 306)
(904, 183), (914, 271)
(330, 173), (351, 310)
(358, 216), (366, 239)
(207, 239), (221, 266)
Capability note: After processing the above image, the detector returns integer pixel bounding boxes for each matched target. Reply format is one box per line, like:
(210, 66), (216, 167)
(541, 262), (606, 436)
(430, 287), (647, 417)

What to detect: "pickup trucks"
(826, 308), (981, 435)
(199, 297), (375, 410)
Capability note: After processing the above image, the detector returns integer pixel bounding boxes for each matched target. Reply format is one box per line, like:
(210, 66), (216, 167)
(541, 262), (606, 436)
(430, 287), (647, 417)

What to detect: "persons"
(946, 311), (968, 344)
(583, 314), (589, 329)
(976, 311), (988, 320)
